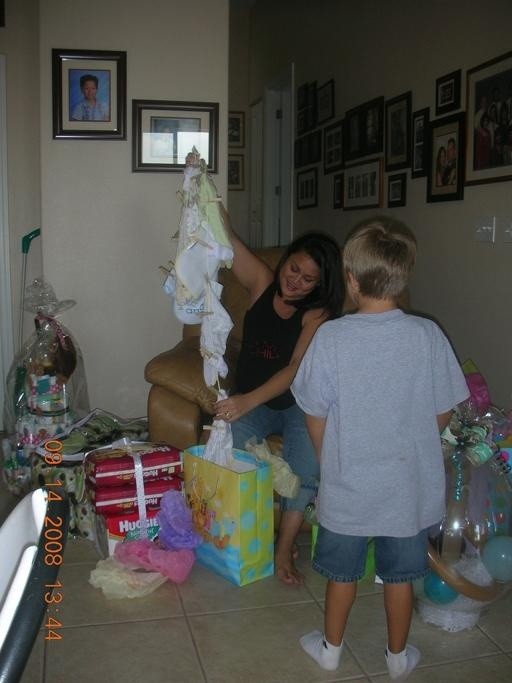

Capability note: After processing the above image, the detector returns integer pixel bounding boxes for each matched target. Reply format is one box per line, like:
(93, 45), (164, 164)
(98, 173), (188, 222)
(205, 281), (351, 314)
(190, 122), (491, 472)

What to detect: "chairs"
(144, 246), (411, 532)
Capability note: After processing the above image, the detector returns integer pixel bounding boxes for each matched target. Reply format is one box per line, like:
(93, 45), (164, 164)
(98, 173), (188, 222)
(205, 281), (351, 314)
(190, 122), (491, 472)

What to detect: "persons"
(153, 126), (176, 156)
(473, 92), (512, 171)
(185, 152), (345, 586)
(288, 217), (471, 683)
(70, 74), (110, 122)
(435, 138), (456, 187)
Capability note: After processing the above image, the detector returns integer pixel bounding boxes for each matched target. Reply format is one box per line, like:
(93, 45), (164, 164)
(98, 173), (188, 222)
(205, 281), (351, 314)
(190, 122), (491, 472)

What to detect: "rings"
(225, 412), (231, 419)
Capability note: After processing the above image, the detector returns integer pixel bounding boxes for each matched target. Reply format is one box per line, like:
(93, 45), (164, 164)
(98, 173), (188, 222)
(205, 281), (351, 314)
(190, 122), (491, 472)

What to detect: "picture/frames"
(294, 50), (512, 211)
(52, 49), (126, 141)
(132, 99), (219, 175)
(229, 154), (245, 191)
(229, 111), (246, 149)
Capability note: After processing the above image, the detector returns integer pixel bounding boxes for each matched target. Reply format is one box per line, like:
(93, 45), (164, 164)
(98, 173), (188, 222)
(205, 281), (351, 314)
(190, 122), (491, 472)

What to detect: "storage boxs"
(88, 503), (171, 560)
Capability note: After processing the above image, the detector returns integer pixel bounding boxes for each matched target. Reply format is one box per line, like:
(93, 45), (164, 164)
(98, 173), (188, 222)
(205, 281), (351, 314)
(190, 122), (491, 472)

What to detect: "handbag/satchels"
(179, 442), (280, 588)
